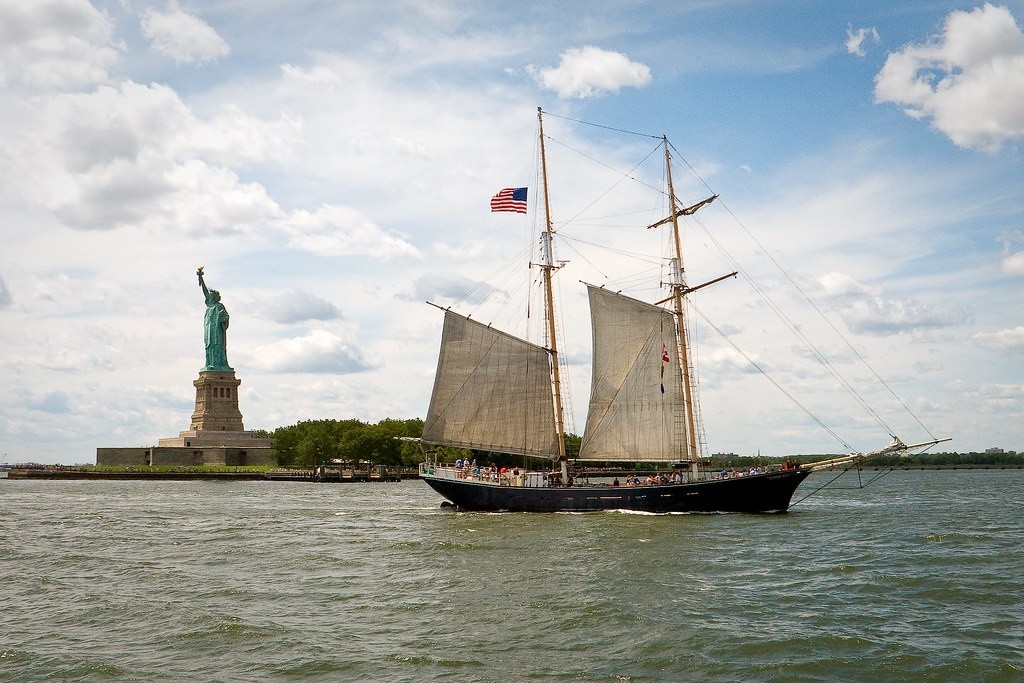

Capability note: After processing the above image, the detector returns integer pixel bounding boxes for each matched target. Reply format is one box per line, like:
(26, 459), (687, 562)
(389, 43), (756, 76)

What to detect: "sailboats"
(389, 106), (956, 523)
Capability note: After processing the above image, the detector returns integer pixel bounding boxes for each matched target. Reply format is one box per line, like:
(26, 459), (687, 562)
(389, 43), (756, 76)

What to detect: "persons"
(500, 467), (507, 473)
(646, 472), (680, 485)
(613, 477), (619, 486)
(716, 463), (787, 480)
(489, 462), (496, 482)
(197, 276), (233, 373)
(512, 466), (519, 475)
(455, 457), (470, 479)
(626, 474), (640, 484)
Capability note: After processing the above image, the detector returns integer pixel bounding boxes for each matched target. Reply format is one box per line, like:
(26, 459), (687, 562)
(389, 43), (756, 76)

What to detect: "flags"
(491, 187), (527, 214)
